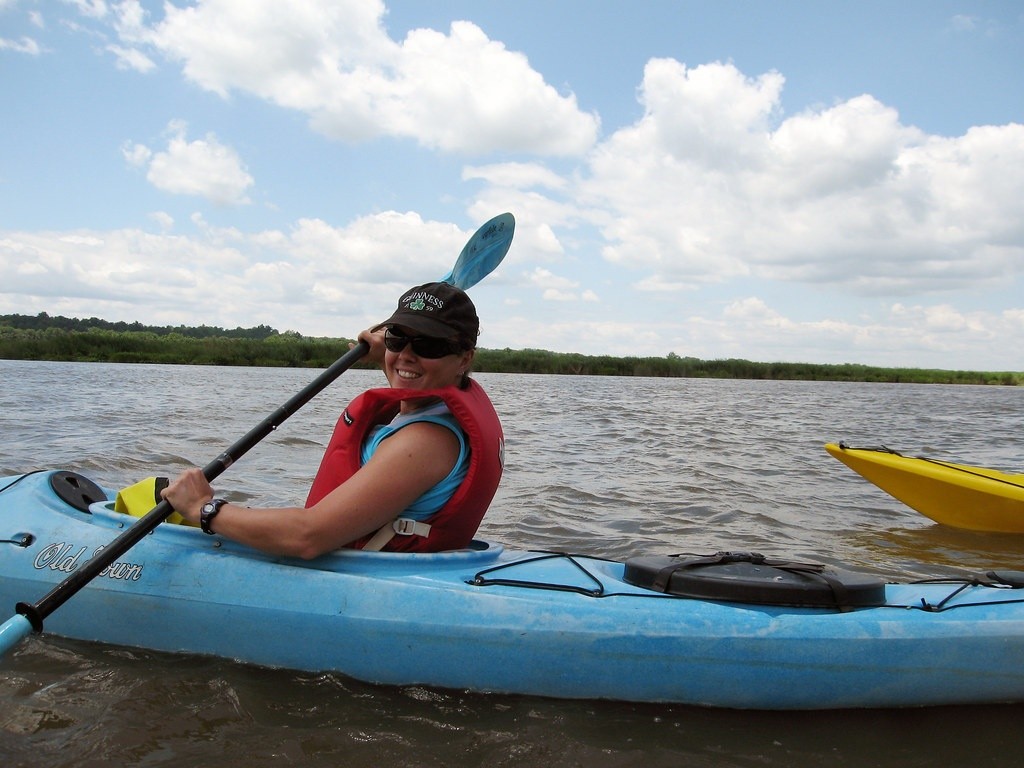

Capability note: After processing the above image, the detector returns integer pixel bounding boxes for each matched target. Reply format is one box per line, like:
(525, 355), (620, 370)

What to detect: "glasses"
(384, 328), (464, 359)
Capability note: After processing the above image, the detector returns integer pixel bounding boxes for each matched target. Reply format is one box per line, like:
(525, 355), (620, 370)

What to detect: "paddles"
(0, 213), (515, 656)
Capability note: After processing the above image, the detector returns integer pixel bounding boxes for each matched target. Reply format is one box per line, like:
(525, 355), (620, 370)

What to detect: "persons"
(160, 283), (505, 560)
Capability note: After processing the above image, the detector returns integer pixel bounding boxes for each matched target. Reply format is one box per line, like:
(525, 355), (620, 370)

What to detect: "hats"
(370, 281), (479, 346)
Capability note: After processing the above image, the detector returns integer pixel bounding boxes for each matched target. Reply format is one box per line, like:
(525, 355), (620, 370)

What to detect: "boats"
(0, 469), (1024, 710)
(821, 440), (1023, 538)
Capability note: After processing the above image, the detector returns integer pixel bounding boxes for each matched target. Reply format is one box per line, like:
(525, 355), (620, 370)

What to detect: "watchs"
(200, 499), (228, 535)
